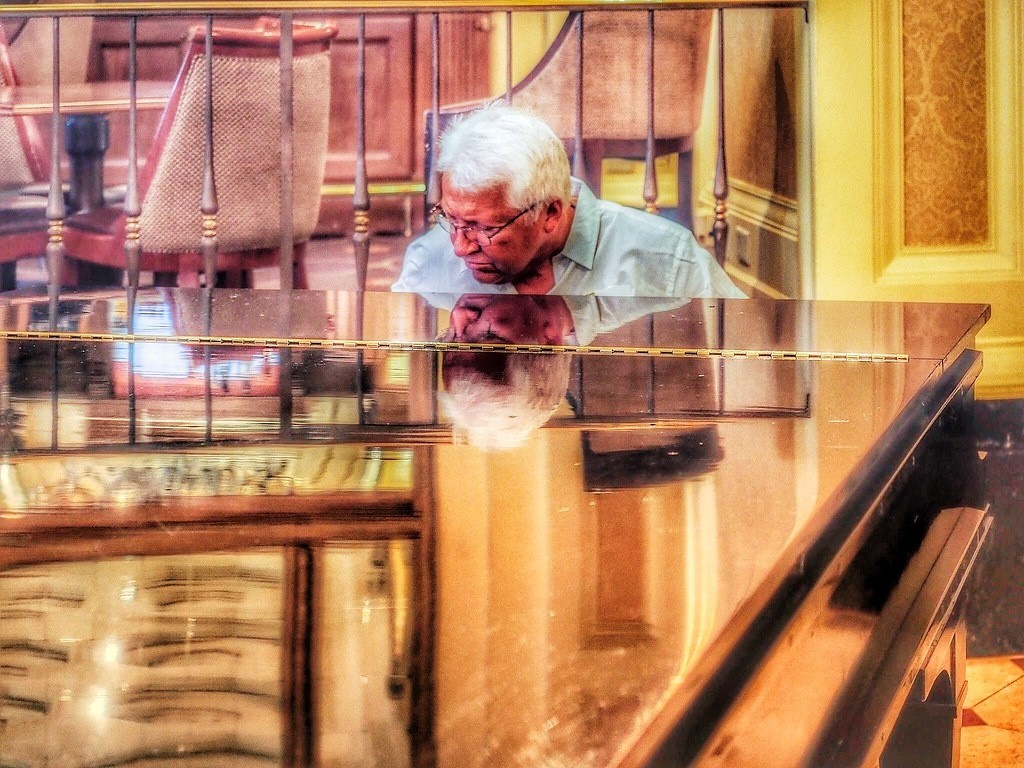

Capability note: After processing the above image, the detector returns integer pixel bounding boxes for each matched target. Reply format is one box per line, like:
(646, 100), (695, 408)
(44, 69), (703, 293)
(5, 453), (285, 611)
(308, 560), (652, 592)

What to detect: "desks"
(0, 81), (172, 286)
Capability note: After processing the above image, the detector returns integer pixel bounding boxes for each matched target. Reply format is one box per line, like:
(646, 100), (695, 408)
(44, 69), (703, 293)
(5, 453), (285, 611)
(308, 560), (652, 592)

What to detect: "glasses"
(434, 329), (513, 344)
(430, 199), (544, 248)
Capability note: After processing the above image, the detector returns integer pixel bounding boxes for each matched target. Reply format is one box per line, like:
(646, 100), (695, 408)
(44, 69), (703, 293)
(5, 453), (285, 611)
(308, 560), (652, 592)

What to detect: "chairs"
(423, 9), (713, 232)
(0, 16), (339, 296)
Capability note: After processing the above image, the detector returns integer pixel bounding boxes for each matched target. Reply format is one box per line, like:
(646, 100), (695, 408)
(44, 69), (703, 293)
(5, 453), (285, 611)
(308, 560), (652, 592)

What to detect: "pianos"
(0, 279), (996, 768)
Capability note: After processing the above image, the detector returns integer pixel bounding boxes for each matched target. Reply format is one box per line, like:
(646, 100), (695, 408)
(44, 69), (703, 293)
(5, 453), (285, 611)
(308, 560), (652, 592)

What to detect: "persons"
(389, 97), (749, 298)
(435, 292), (692, 450)
